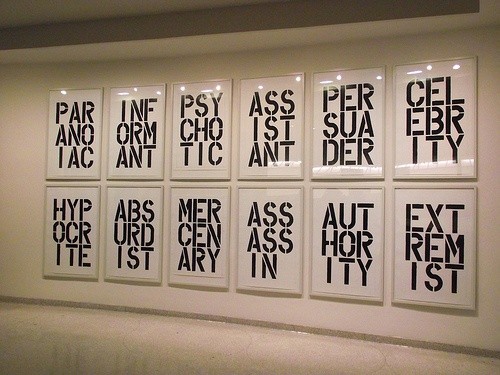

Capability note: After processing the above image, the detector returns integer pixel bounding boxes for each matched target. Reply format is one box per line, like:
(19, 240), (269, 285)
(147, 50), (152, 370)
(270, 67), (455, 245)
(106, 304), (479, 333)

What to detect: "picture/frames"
(309, 183), (384, 304)
(238, 181), (304, 297)
(105, 183), (163, 287)
(108, 85), (166, 181)
(170, 80), (231, 181)
(392, 183), (476, 311)
(310, 65), (386, 180)
(239, 72), (304, 181)
(393, 56), (477, 180)
(170, 185), (230, 293)
(43, 182), (98, 281)
(47, 89), (103, 180)
